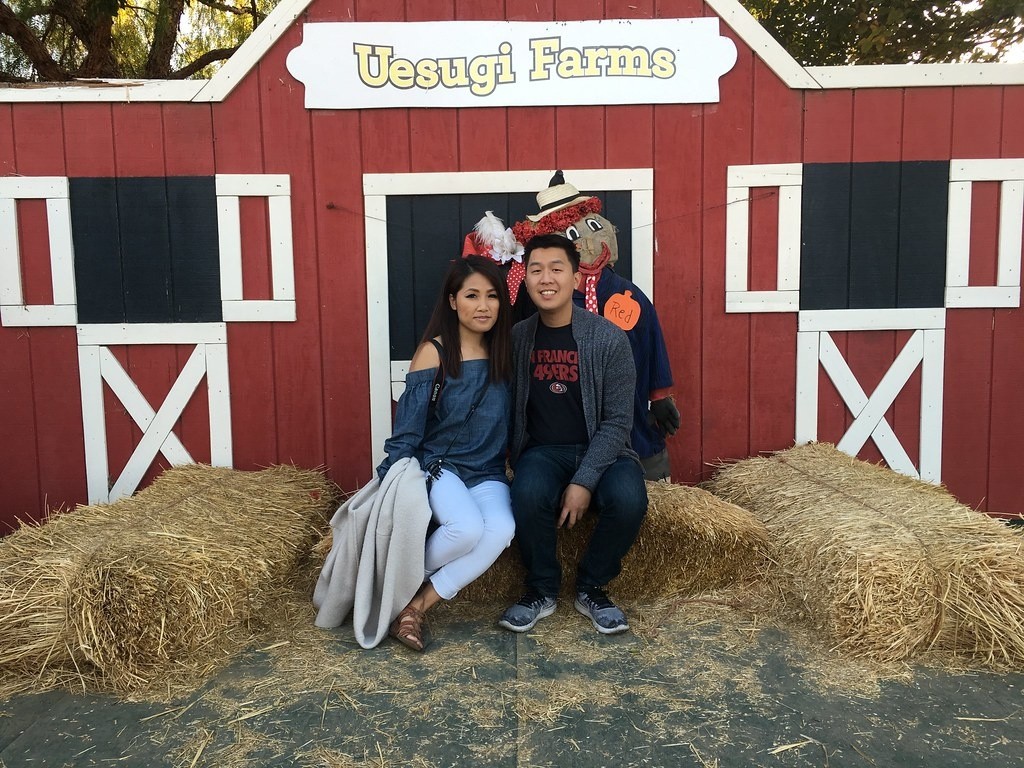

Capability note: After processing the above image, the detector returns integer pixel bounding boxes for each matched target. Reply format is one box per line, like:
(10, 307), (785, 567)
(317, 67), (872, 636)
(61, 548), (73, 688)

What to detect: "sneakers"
(574, 585), (630, 634)
(497, 592), (558, 634)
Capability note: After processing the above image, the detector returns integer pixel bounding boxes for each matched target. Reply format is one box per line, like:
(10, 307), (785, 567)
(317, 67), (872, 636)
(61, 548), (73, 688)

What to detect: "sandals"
(388, 592), (426, 651)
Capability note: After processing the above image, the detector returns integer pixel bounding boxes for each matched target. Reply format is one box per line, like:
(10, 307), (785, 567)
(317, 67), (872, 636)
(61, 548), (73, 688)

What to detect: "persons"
(375, 252), (517, 653)
(462, 169), (682, 482)
(499, 232), (650, 636)
